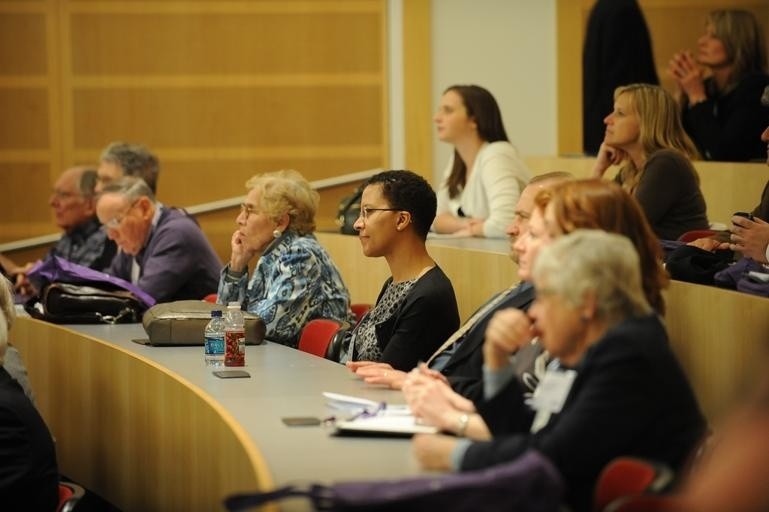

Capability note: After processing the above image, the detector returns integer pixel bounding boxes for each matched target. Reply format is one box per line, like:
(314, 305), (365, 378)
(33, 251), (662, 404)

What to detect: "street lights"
(25, 280), (142, 325)
(144, 300), (266, 346)
(336, 179), (368, 235)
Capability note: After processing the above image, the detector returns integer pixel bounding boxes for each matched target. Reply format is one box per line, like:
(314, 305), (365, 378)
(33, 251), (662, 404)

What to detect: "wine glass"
(381, 367), (389, 378)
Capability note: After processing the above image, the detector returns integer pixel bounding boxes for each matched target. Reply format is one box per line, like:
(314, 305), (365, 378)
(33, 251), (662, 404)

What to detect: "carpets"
(426, 280), (526, 365)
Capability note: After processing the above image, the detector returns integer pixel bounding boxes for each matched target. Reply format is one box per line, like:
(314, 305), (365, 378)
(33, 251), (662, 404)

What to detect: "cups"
(240, 203), (278, 219)
(100, 201), (139, 232)
(52, 188), (92, 200)
(360, 207), (409, 220)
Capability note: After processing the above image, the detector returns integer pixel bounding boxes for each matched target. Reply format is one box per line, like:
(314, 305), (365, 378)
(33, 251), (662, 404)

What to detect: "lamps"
(596, 460), (656, 508)
(57, 482), (85, 512)
(298, 319), (342, 358)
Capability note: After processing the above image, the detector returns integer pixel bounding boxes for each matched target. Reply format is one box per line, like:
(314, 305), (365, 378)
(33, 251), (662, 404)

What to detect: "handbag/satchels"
(457, 410), (469, 438)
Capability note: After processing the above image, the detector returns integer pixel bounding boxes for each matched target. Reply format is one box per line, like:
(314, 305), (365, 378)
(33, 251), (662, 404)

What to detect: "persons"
(0, 309), (59, 512)
(592, 85), (711, 241)
(99, 178), (226, 307)
(17, 163), (118, 303)
(514, 182), (558, 283)
(427, 84), (530, 238)
(94, 140), (156, 203)
(687, 84), (769, 297)
(217, 172), (350, 349)
(412, 229), (709, 501)
(2, 274), (36, 408)
(673, 8), (768, 162)
(344, 174), (578, 406)
(402, 176), (666, 442)
(338, 169), (463, 376)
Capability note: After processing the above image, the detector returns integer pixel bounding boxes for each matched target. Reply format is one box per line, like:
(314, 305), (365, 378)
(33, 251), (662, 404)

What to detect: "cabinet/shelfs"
(224, 301), (245, 368)
(204, 310), (226, 371)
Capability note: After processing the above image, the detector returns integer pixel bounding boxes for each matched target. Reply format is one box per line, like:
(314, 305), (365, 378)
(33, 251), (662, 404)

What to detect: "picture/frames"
(311, 232), (769, 507)
(2, 314), (448, 510)
